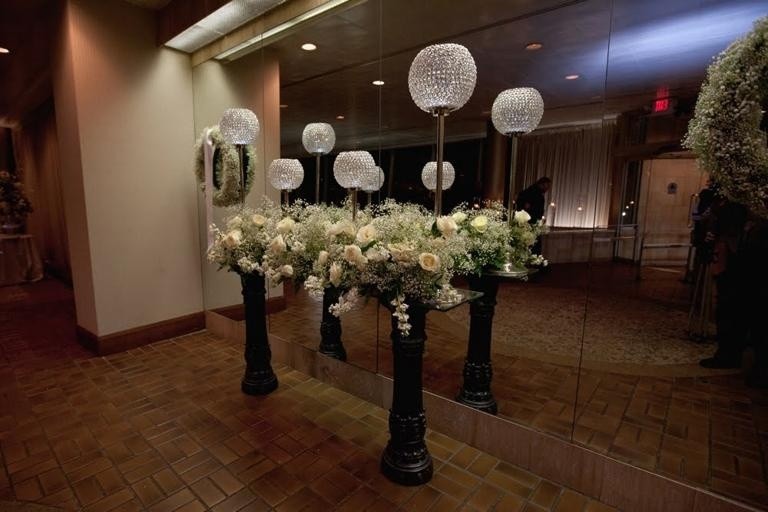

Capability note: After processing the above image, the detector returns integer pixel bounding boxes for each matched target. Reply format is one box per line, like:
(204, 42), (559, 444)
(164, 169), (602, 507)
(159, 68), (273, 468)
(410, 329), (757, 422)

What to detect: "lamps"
(269, 159), (303, 208)
(424, 162), (455, 217)
(492, 88), (545, 226)
(219, 107), (259, 211)
(408, 43), (478, 215)
(302, 123), (337, 205)
(361, 165), (385, 205)
(333, 150), (375, 224)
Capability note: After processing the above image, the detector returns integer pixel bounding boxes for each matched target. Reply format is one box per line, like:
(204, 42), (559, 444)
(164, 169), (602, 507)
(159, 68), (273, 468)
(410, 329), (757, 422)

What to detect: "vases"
(2, 225), (23, 234)
(383, 286), (484, 485)
(458, 266), (540, 416)
(239, 270), (278, 395)
(319, 283), (348, 364)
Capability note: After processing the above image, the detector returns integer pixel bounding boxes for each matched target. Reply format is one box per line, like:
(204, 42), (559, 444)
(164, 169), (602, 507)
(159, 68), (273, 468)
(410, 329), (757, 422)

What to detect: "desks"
(0, 232), (45, 285)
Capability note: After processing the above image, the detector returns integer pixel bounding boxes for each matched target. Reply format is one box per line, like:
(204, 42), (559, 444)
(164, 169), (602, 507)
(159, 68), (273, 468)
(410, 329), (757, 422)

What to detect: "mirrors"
(192, 0), (768, 511)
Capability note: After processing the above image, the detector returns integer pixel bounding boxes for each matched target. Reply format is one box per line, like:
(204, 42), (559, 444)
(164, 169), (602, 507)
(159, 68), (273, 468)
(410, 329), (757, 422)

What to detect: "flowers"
(229, 144), (257, 207)
(680, 14), (768, 216)
(0, 171), (33, 224)
(192, 128), (240, 208)
(295, 199), (548, 280)
(208, 197), (461, 341)
